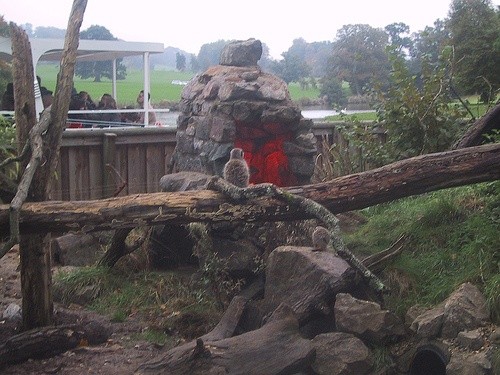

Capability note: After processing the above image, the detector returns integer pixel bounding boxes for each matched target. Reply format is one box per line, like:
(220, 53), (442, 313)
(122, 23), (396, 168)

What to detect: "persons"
(3, 75), (139, 127)
(133, 89), (156, 128)
